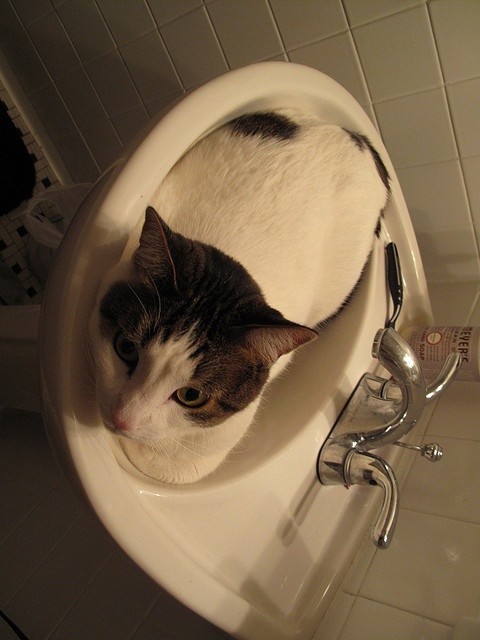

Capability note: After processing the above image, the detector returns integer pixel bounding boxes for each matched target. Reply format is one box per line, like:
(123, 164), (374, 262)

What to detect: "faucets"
(316, 326), (463, 550)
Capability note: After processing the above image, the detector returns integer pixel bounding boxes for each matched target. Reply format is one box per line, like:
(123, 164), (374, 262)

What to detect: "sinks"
(37, 60), (437, 640)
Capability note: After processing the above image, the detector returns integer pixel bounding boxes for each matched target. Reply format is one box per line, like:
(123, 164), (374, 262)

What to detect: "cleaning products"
(403, 322), (480, 385)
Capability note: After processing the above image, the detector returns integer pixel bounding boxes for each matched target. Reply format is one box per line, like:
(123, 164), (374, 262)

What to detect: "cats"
(69, 102), (395, 489)
